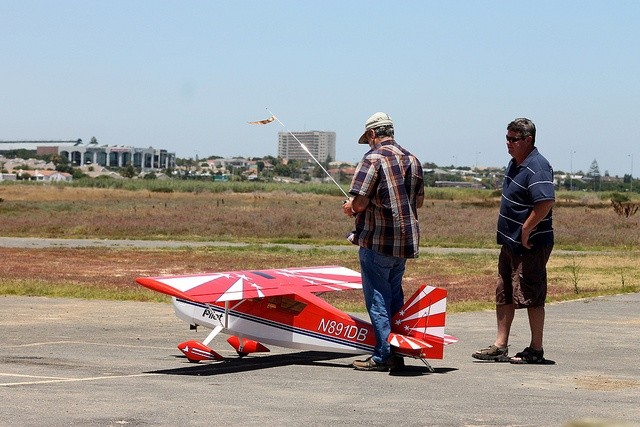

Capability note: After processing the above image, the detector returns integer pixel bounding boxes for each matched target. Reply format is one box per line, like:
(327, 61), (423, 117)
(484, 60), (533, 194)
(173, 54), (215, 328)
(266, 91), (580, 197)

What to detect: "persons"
(342, 111), (424, 374)
(472, 118), (555, 362)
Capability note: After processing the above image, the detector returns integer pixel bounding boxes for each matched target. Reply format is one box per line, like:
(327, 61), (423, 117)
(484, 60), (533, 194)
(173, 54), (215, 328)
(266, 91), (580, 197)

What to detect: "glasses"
(506, 134), (528, 142)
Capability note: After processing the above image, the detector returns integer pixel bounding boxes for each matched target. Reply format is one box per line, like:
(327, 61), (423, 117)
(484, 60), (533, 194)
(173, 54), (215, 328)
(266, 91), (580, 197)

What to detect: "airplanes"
(135, 265), (459, 372)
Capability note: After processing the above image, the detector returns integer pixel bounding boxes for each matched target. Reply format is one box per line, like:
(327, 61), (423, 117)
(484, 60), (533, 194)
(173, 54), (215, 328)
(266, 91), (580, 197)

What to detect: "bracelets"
(350, 205), (356, 214)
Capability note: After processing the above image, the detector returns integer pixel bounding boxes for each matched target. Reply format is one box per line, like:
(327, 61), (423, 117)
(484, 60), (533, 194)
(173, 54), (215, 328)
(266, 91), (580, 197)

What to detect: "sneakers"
(393, 357), (406, 371)
(353, 357), (391, 372)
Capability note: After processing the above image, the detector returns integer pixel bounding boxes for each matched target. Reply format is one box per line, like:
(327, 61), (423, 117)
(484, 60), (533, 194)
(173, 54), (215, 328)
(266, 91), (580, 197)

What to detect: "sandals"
(471, 344), (510, 362)
(509, 346), (546, 364)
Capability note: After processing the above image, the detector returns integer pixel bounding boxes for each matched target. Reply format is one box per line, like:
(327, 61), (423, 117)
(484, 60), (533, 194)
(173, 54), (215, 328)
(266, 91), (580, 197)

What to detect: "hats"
(359, 112), (393, 143)
